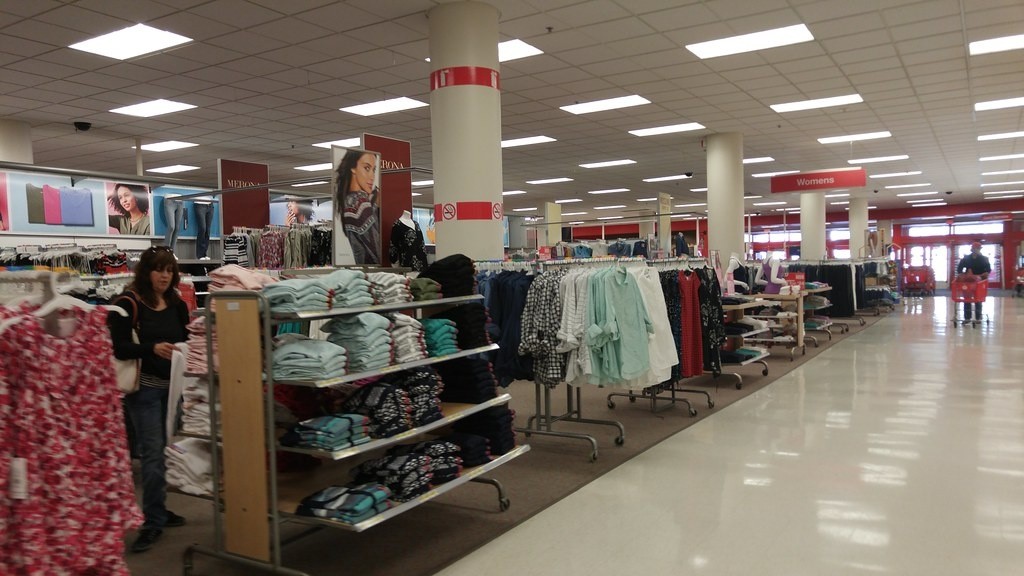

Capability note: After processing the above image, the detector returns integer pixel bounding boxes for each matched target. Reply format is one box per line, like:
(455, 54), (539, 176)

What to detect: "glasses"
(152, 245), (170, 253)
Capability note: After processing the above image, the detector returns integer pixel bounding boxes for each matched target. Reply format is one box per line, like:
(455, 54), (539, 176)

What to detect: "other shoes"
(963, 320), (970, 324)
(976, 321), (981, 324)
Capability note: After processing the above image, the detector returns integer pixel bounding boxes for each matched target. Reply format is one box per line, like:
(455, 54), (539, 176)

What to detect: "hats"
(972, 242), (981, 248)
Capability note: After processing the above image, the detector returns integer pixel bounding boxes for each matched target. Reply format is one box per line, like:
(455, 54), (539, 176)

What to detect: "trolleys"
(951, 274), (989, 328)
(1010, 266), (1024, 299)
(902, 265), (937, 296)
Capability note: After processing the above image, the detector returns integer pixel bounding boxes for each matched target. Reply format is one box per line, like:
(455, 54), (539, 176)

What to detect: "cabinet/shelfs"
(719, 281), (836, 364)
(157, 287), (531, 535)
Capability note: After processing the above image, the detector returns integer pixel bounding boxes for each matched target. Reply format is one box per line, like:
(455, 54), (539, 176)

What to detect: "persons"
(105, 245), (191, 551)
(675, 232), (690, 258)
(725, 252), (749, 295)
(754, 250), (785, 293)
(389, 210), (429, 272)
(957, 240), (991, 325)
(163, 193), (183, 260)
(334, 150), (381, 265)
(194, 197), (214, 260)
(107, 183), (150, 236)
(286, 199), (315, 227)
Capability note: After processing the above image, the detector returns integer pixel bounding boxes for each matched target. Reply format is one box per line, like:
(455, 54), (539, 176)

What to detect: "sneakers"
(131, 523), (163, 550)
(162, 510), (185, 526)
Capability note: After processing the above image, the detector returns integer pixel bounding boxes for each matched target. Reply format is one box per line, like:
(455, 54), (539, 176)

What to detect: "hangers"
(0, 242), (143, 339)
(555, 236), (647, 244)
(228, 219), (331, 236)
(474, 255), (715, 275)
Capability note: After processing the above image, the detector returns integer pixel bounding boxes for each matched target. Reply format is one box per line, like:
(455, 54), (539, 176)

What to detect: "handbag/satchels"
(111, 291), (142, 392)
